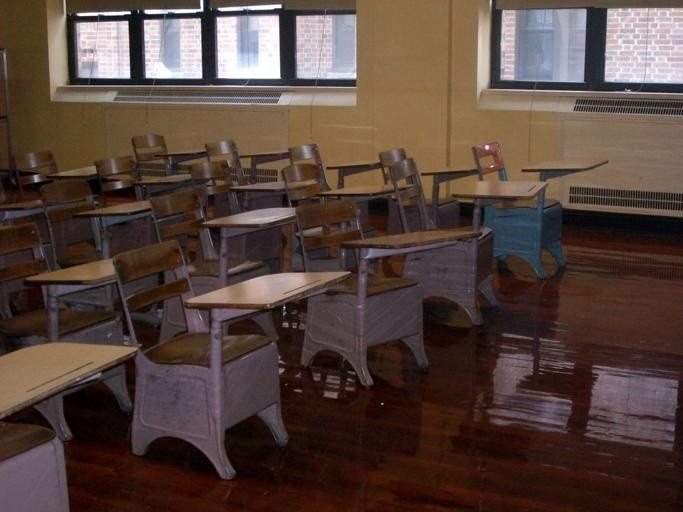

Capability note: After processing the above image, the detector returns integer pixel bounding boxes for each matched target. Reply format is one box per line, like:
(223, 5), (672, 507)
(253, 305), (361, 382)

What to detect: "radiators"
(562, 93), (683, 220)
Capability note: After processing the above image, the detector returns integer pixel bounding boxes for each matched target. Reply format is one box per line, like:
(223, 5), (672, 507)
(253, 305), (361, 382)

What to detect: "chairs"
(0, 130), (611, 512)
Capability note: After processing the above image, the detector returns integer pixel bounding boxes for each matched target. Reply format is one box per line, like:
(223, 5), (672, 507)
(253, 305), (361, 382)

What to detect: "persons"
(523, 46), (551, 77)
